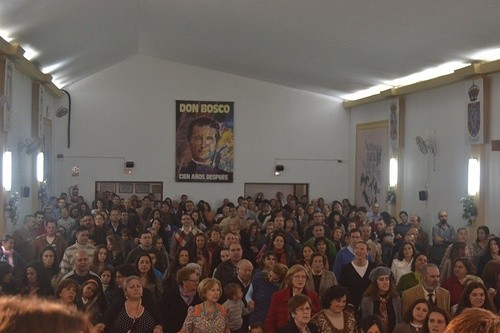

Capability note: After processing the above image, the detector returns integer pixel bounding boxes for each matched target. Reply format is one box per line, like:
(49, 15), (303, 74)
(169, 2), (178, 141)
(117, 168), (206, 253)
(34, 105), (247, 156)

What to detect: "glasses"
(184, 278), (200, 285)
(295, 307), (311, 313)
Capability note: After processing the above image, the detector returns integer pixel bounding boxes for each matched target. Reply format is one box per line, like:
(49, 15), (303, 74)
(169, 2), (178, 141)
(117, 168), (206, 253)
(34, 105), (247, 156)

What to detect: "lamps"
(125, 162), (134, 168)
(275, 165), (284, 171)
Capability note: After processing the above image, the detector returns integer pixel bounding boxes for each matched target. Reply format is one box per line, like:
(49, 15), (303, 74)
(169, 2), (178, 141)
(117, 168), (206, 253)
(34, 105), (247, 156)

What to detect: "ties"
(427, 293), (434, 305)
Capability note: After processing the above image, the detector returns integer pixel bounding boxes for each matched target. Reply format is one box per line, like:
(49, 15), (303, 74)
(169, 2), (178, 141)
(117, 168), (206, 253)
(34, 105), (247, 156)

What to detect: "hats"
(369, 266), (391, 282)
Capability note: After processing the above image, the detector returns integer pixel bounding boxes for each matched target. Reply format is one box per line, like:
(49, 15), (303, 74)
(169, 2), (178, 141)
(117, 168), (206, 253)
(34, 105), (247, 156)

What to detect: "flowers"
(459, 195), (478, 228)
(385, 188), (396, 204)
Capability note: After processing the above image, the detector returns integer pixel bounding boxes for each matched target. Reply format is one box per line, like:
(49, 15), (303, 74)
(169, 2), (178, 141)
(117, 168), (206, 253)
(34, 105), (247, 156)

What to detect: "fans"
(416, 136), (437, 156)
(45, 102), (68, 119)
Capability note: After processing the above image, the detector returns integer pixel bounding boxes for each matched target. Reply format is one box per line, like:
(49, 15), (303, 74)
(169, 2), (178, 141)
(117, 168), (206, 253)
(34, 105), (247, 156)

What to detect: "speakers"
(125, 162), (133, 167)
(276, 165), (284, 171)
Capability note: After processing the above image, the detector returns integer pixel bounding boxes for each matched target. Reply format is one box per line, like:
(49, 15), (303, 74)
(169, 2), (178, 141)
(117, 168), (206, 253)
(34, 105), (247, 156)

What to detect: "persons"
(0, 187), (500, 333)
